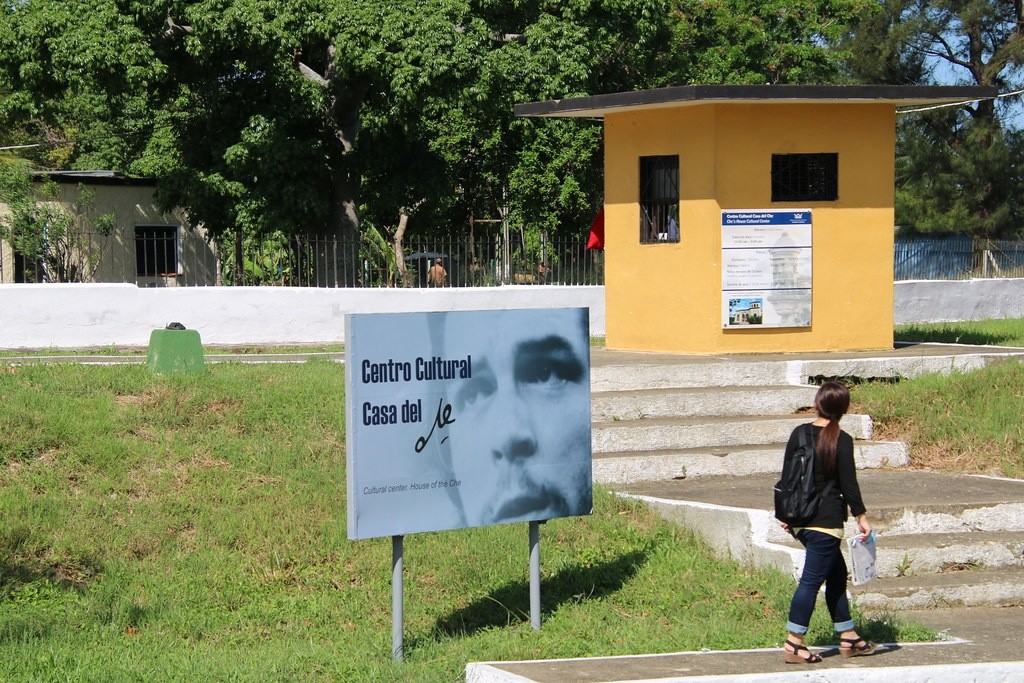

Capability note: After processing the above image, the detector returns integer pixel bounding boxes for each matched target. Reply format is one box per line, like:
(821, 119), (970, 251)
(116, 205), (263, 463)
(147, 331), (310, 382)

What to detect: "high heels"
(839, 636), (878, 658)
(785, 639), (824, 663)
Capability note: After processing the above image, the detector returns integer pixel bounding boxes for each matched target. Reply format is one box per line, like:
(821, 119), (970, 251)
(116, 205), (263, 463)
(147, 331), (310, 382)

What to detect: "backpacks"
(775, 425), (825, 523)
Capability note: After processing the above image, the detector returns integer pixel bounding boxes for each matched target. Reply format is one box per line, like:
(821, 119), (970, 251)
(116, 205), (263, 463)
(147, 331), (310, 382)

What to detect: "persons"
(779, 381), (877, 664)
(426, 307), (593, 528)
